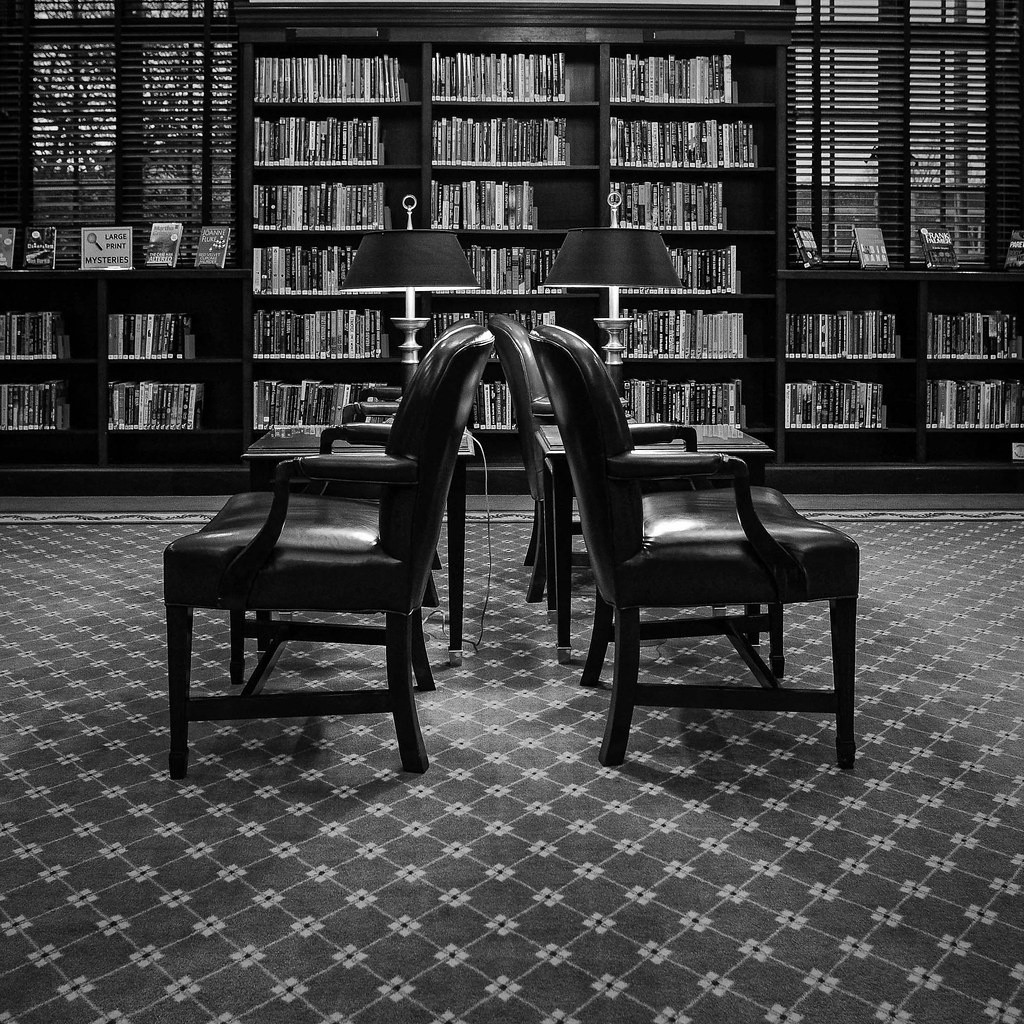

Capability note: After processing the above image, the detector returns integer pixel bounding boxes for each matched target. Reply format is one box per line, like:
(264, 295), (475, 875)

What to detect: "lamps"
(541, 193), (687, 416)
(340, 193), (481, 399)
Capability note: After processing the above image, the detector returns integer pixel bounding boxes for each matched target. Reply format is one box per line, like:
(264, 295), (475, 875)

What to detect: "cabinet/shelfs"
(0, 2), (1024, 496)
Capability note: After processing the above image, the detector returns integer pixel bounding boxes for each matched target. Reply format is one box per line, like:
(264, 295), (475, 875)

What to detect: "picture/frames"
(80, 226), (134, 271)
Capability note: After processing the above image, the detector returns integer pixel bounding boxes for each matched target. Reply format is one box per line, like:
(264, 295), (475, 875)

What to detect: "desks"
(242, 423), (472, 667)
(532, 424), (775, 667)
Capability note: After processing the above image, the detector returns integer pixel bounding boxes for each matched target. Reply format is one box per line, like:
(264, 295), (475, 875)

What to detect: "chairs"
(164, 315), (862, 781)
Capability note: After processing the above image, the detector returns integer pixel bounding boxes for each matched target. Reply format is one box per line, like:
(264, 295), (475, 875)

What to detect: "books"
(0, 227), (16, 269)
(918, 228), (960, 269)
(23, 225), (57, 269)
(253, 305), (1024, 454)
(853, 227), (890, 269)
(791, 226), (823, 265)
(145, 222), (183, 268)
(1004, 230), (1024, 268)
(0, 311), (201, 429)
(252, 179), (743, 292)
(193, 225), (232, 268)
(250, 51), (766, 164)
